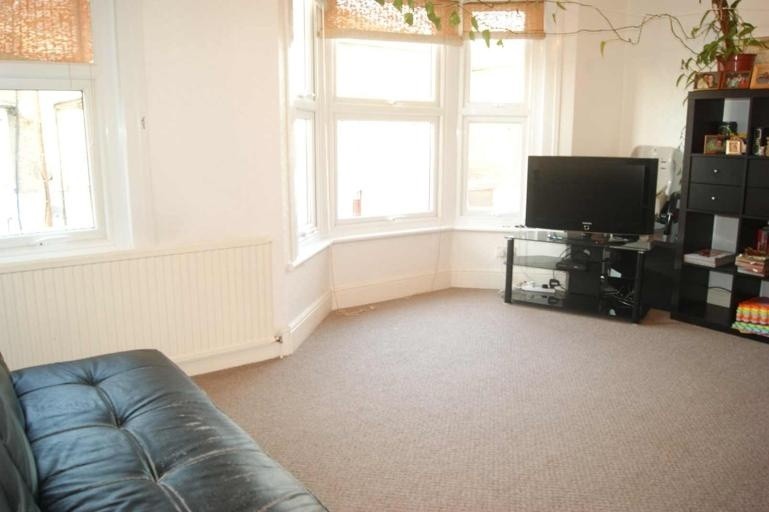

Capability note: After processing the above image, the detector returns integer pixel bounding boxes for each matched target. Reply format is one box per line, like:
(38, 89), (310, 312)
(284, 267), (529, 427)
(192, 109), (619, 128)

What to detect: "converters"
(550, 279), (560, 288)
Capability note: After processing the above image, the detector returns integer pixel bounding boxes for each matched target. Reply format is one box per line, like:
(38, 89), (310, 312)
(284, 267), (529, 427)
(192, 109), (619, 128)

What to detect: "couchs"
(0, 342), (332, 511)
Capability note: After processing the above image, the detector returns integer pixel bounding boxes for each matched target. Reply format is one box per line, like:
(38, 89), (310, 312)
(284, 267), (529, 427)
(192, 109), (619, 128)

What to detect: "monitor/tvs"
(526, 156), (658, 243)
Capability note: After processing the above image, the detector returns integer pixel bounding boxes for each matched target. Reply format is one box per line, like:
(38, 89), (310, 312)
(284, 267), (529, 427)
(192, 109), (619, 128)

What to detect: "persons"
(710, 139), (724, 153)
(696, 71), (750, 89)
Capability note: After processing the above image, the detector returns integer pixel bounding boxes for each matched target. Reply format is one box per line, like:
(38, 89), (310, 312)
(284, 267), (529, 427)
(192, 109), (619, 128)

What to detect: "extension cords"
(521, 284), (556, 293)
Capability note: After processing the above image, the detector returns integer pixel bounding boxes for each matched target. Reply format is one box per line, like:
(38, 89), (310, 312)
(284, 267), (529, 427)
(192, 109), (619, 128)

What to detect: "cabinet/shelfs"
(641, 228), (674, 312)
(669, 87), (769, 347)
(496, 227), (654, 327)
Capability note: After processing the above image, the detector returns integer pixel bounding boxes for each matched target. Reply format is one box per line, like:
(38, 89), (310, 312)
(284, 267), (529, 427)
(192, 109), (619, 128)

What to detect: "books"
(684, 249), (768, 277)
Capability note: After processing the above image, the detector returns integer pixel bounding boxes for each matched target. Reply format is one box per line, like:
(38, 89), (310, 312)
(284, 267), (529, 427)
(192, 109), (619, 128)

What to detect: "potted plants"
(370, 1), (769, 80)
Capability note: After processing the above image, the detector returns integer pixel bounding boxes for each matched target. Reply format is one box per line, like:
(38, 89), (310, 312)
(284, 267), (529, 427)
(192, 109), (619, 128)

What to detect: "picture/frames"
(748, 62), (769, 89)
(720, 71), (752, 90)
(694, 70), (722, 91)
